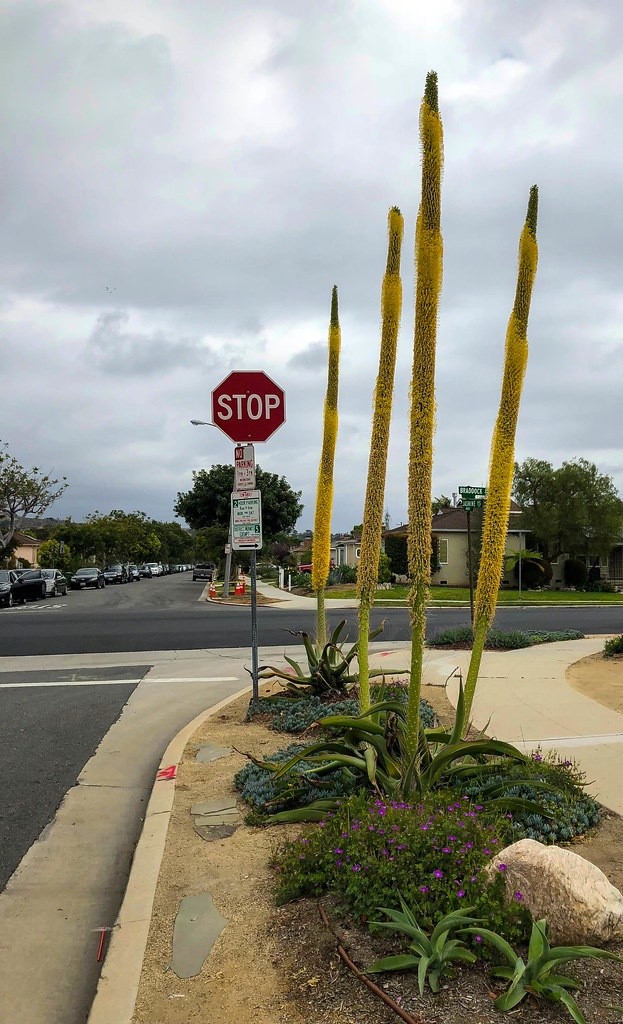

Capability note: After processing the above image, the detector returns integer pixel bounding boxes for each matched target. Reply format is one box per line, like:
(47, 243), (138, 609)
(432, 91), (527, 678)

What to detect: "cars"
(104, 562), (195, 585)
(0, 569), (43, 607)
(38, 569), (68, 597)
(70, 567), (107, 590)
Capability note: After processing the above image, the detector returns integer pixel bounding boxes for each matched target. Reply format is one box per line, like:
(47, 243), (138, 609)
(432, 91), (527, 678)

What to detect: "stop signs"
(209, 371), (288, 443)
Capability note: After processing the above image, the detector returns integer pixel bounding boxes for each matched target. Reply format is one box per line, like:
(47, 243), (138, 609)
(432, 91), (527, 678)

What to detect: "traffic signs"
(232, 445), (257, 491)
(462, 499), (485, 508)
(458, 486), (486, 495)
(230, 489), (263, 551)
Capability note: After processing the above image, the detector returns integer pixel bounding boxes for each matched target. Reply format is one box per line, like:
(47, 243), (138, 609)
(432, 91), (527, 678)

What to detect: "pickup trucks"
(297, 558), (337, 575)
(192, 563), (214, 582)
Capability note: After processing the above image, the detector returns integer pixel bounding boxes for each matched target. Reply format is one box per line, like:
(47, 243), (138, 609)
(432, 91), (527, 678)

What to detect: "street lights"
(190, 419), (241, 599)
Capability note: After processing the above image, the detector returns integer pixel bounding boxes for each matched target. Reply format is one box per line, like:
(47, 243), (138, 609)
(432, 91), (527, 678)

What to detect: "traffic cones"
(208, 576), (246, 599)
